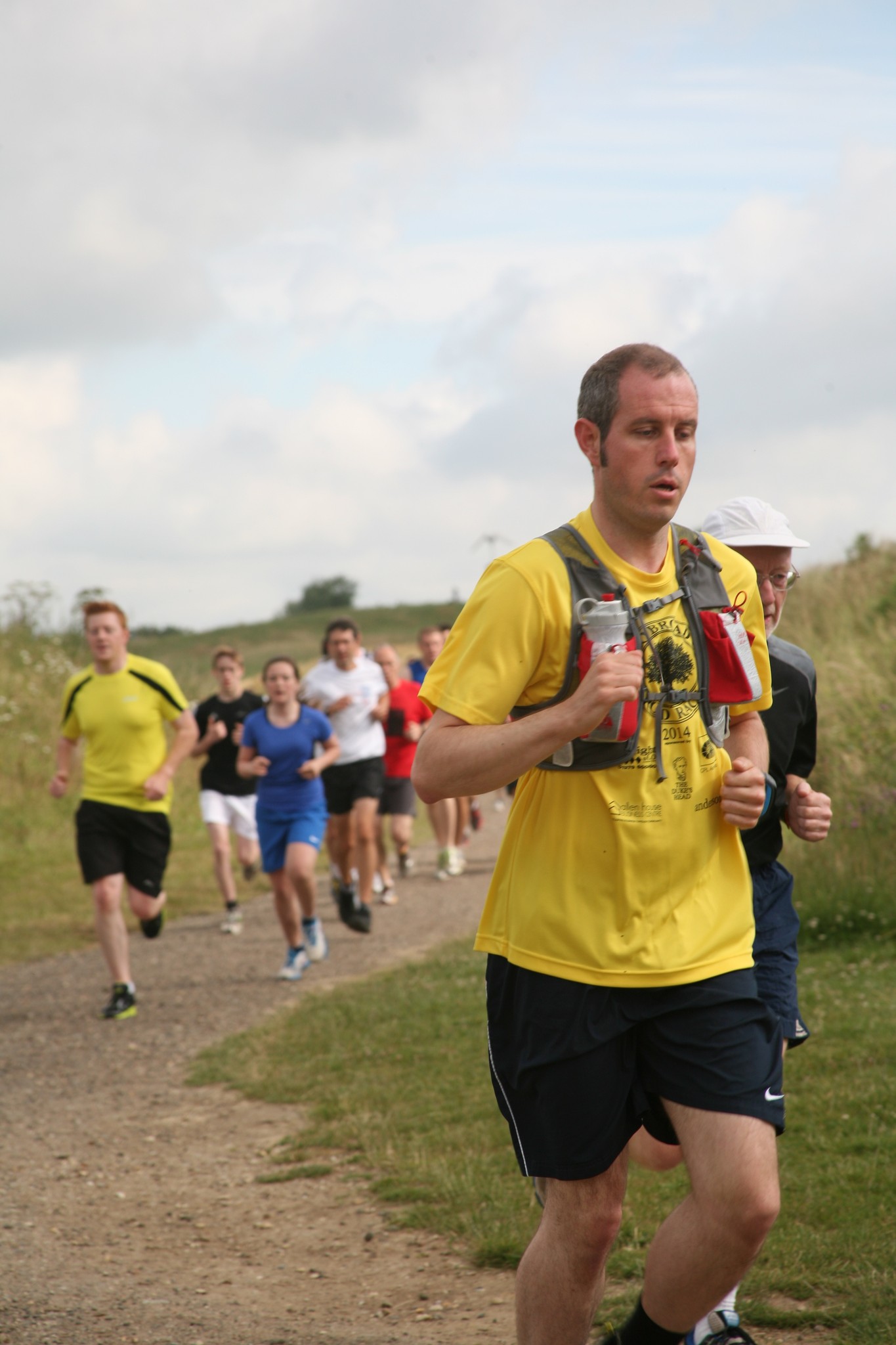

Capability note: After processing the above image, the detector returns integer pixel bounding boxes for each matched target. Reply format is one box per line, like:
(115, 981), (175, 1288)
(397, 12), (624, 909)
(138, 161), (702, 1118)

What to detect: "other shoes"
(382, 890), (397, 903)
(685, 1309), (756, 1344)
(221, 914), (241, 931)
(349, 911), (369, 931)
(334, 886), (354, 921)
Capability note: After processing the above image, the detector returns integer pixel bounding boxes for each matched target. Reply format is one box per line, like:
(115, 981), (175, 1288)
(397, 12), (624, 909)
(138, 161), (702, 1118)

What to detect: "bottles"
(574, 592), (630, 644)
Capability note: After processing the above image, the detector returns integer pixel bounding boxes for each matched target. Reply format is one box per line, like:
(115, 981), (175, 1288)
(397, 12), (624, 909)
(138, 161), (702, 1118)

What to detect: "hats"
(697, 495), (808, 549)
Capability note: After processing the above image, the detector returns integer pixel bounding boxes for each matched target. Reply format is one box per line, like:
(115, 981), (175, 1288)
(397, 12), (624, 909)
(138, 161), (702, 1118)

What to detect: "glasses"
(755, 563), (801, 591)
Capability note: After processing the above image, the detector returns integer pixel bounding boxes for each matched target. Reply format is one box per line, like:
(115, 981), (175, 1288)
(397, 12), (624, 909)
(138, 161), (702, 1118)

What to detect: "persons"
(192, 620), (483, 978)
(412, 343), (834, 1344)
(48, 602), (201, 1022)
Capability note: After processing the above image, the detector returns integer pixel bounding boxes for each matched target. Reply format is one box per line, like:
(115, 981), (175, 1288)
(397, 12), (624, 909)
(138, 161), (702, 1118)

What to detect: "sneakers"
(301, 919), (326, 961)
(279, 949), (309, 979)
(99, 984), (138, 1019)
(140, 909), (163, 936)
